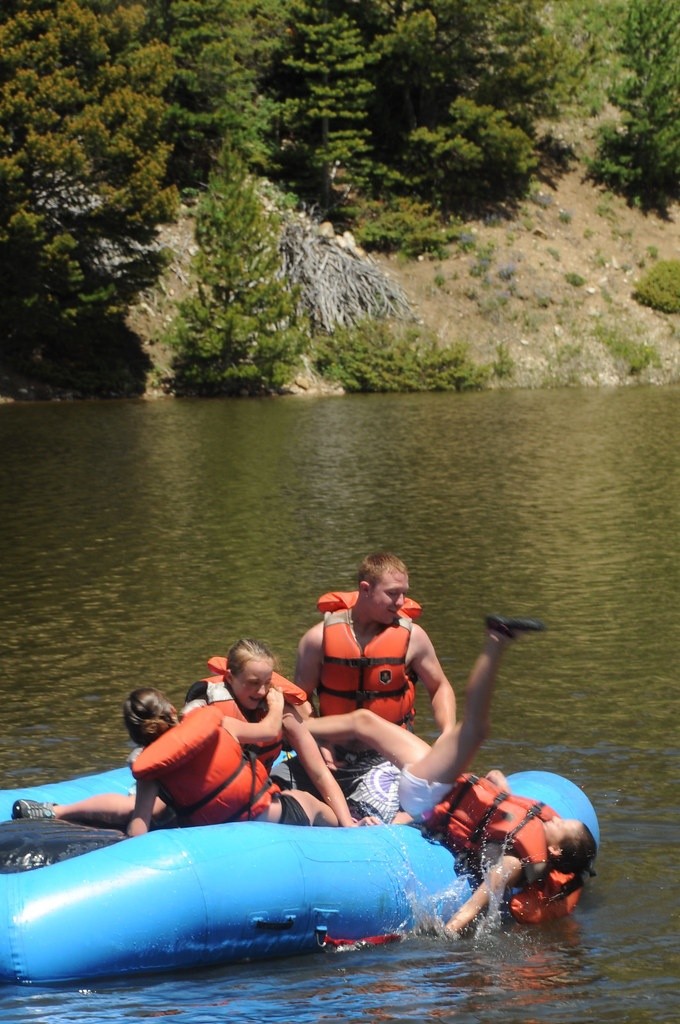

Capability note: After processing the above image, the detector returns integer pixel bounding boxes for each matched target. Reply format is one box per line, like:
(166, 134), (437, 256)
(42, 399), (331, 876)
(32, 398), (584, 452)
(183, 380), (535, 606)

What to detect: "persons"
(277, 614), (598, 936)
(126, 687), (340, 840)
(12, 638), (386, 830)
(292, 552), (457, 827)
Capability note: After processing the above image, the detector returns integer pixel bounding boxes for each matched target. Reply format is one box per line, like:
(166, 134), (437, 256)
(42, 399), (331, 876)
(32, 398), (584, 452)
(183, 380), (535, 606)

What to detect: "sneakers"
(11, 799), (57, 821)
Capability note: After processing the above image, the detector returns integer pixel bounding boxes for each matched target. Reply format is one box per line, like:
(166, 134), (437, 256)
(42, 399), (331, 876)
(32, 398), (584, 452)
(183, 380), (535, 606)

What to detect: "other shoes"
(484, 613), (544, 649)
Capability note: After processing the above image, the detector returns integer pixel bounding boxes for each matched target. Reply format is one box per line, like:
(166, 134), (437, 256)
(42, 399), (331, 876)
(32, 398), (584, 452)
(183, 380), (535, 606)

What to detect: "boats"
(0, 757), (599, 988)
